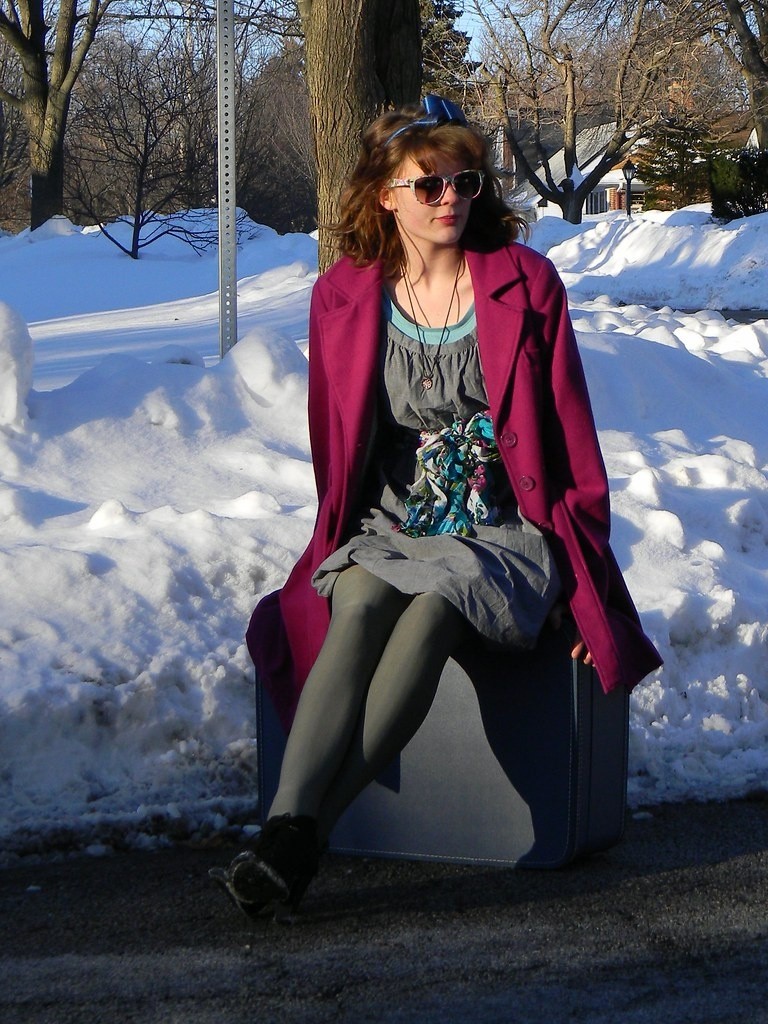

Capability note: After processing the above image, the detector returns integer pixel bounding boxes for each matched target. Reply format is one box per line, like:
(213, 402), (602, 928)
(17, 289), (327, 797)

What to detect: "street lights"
(622, 159), (636, 216)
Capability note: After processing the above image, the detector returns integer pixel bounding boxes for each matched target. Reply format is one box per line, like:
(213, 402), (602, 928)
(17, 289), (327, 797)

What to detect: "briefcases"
(256, 628), (629, 869)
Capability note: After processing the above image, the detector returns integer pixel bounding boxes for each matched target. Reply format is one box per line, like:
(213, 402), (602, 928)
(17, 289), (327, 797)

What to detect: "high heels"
(209, 814), (330, 923)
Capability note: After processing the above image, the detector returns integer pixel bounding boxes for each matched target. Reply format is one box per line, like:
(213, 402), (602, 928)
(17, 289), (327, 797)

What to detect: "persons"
(214, 97), (666, 923)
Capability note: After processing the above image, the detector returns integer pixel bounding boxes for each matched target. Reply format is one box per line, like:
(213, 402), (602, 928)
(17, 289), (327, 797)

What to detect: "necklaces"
(398, 260), (460, 390)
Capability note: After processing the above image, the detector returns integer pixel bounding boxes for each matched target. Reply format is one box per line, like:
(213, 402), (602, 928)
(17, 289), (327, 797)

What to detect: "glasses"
(389, 169), (485, 205)
